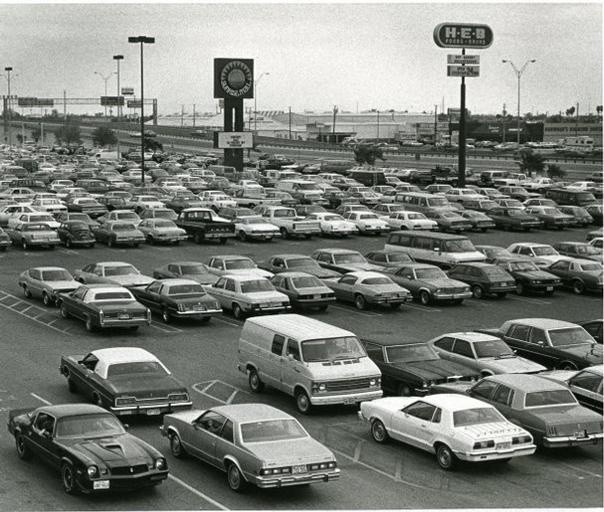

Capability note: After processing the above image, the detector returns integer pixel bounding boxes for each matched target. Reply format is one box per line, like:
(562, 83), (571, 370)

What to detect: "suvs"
(553, 242), (603, 260)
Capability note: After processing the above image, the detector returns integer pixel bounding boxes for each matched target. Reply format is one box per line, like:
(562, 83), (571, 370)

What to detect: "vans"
(237, 312), (384, 414)
(384, 228), (488, 271)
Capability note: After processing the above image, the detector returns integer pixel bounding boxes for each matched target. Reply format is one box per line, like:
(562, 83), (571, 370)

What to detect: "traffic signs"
(447, 54), (481, 64)
(447, 65), (481, 78)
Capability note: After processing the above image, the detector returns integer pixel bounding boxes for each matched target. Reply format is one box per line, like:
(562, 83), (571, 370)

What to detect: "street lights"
(254, 71), (271, 128)
(126, 35), (155, 185)
(248, 111), (259, 129)
(94, 70), (117, 118)
(113, 54), (124, 121)
(502, 57), (537, 147)
(4, 66), (12, 124)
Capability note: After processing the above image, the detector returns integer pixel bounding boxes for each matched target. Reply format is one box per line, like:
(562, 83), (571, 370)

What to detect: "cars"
(325, 271), (413, 312)
(538, 257), (603, 295)
(7, 403), (170, 497)
(56, 283), (152, 332)
(474, 244), (512, 265)
(256, 253), (343, 281)
(267, 271), (336, 312)
(0, 140), (603, 249)
(154, 403), (340, 497)
(308, 248), (384, 275)
(506, 242), (562, 263)
(203, 254), (275, 280)
(61, 345), (194, 420)
(202, 274), (292, 318)
(445, 262), (518, 300)
(72, 261), (157, 291)
(490, 256), (563, 297)
(358, 314), (604, 474)
(364, 249), (417, 270)
(154, 260), (220, 287)
(129, 278), (223, 324)
(18, 266), (85, 307)
(386, 263), (473, 307)
